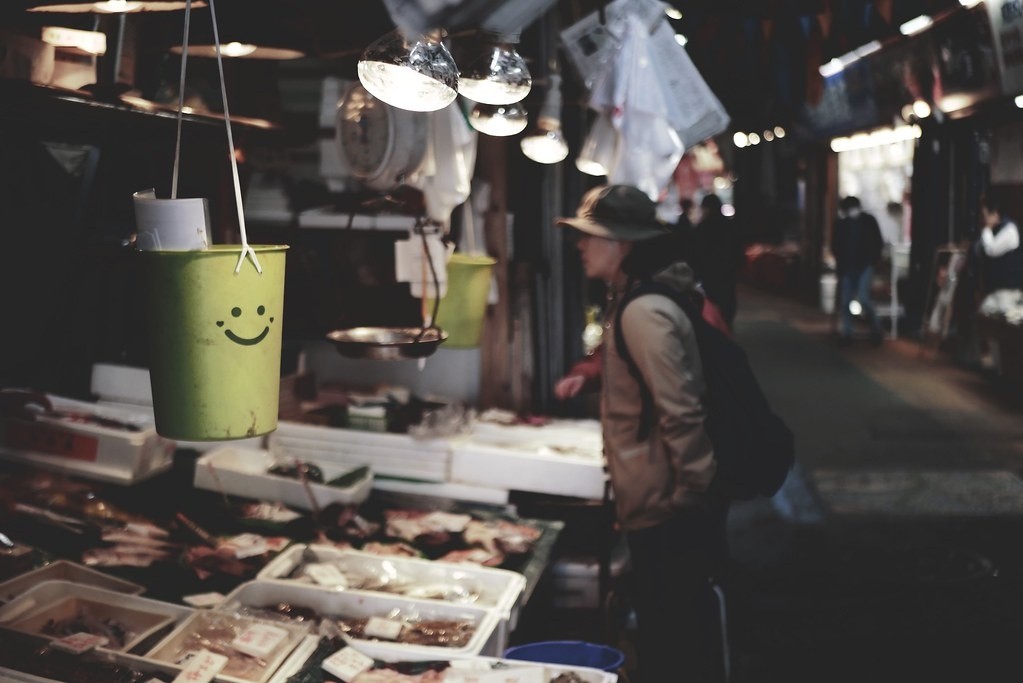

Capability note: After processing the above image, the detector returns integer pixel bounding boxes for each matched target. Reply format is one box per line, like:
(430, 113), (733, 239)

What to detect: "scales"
(322, 82), (450, 363)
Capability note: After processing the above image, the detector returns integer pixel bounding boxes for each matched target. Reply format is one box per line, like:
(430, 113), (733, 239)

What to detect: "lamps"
(520, 129), (569, 164)
(457, 32), (532, 106)
(358, 28), (458, 112)
(470, 104), (529, 137)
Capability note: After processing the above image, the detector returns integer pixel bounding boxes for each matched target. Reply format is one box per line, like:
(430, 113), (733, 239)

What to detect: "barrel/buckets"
(122, 227), (294, 443)
(417, 253), (497, 350)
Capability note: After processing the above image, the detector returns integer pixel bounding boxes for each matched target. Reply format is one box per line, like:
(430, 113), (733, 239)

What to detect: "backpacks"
(614, 283), (793, 498)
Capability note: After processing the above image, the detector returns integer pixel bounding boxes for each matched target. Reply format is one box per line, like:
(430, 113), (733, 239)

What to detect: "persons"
(671, 192), (742, 334)
(548, 184), (741, 683)
(830, 196), (890, 351)
(976, 198), (1023, 371)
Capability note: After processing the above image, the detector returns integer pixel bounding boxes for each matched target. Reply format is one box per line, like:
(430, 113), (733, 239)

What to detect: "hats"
(554, 184), (672, 244)
